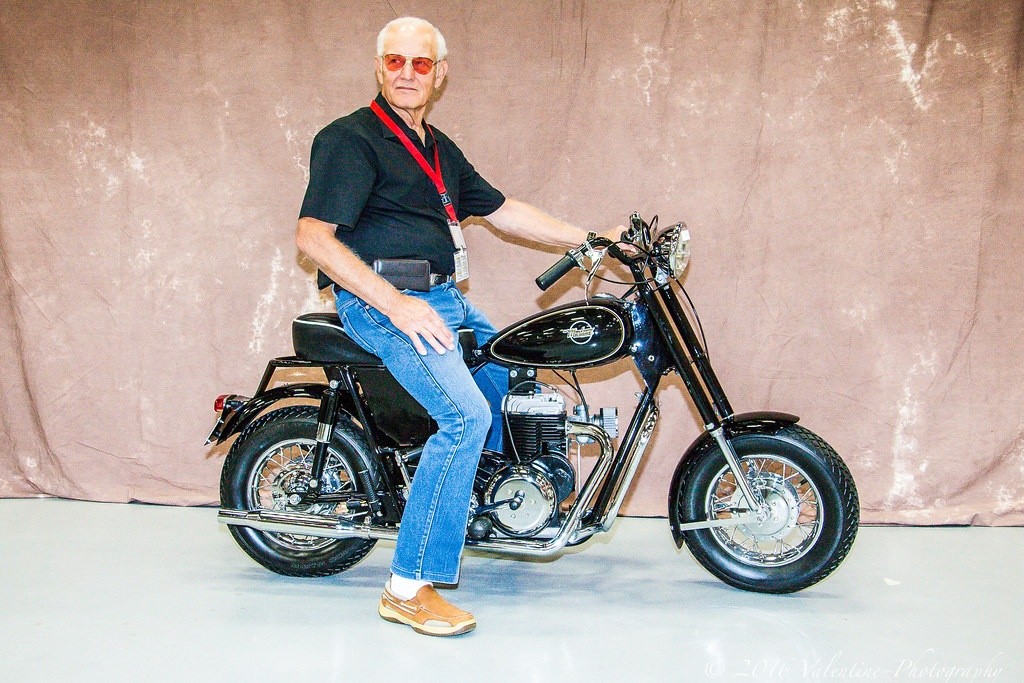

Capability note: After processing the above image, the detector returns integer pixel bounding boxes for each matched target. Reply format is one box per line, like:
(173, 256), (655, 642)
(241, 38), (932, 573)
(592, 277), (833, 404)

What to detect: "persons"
(295, 17), (637, 636)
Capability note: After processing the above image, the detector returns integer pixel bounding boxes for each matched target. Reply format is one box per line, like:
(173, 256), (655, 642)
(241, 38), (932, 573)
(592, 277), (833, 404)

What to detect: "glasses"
(380, 52), (443, 75)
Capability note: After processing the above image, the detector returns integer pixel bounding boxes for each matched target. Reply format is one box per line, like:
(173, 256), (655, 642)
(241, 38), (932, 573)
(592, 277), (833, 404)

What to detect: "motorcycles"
(208, 211), (860, 595)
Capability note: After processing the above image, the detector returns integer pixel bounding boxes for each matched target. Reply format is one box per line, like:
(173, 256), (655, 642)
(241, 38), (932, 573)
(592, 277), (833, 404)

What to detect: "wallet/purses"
(376, 260), (433, 291)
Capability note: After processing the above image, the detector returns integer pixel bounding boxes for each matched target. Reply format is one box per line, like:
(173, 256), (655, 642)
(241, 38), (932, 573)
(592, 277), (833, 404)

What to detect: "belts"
(432, 271), (461, 285)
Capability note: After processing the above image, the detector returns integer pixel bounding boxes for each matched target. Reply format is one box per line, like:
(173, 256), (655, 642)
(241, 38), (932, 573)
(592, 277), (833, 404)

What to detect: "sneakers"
(381, 578), (478, 636)
